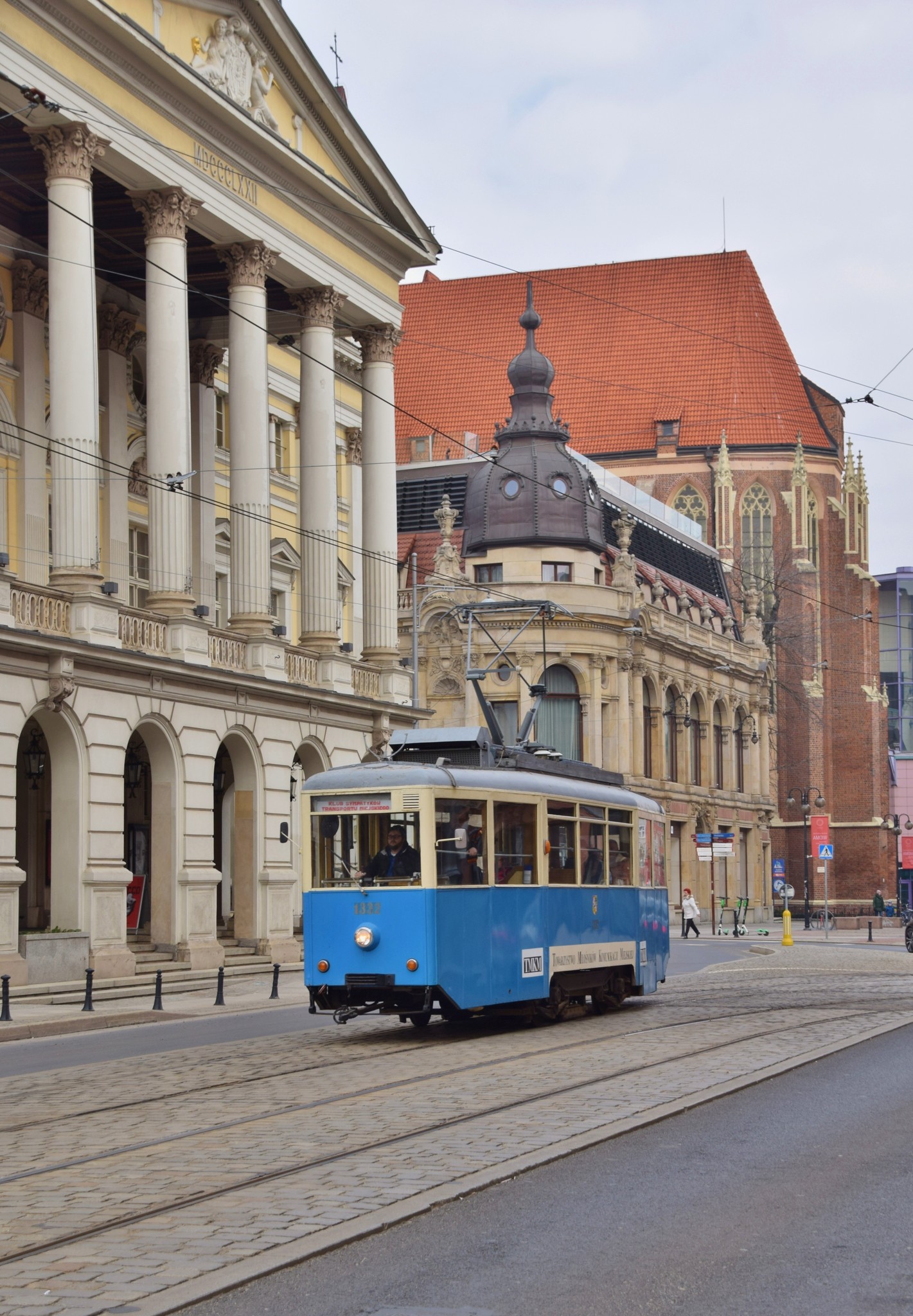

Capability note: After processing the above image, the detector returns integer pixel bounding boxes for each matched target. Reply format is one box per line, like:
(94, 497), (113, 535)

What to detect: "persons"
(883, 901), (896, 917)
(498, 856), (512, 885)
(436, 805), (482, 884)
(355, 825), (421, 887)
(581, 848), (603, 885)
(682, 888), (700, 939)
(873, 890), (884, 916)
(609, 839), (631, 886)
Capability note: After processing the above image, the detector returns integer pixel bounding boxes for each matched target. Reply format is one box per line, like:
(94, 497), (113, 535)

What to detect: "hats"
(876, 890), (881, 894)
(888, 901), (892, 904)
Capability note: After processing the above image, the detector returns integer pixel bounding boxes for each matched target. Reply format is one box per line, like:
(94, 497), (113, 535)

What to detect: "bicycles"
(895, 902), (912, 925)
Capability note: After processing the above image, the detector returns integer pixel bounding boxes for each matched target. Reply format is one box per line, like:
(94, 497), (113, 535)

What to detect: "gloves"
(873, 907), (875, 910)
(894, 913), (896, 915)
(883, 909), (885, 911)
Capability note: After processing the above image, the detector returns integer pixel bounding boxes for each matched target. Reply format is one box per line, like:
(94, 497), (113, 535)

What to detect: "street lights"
(786, 787), (826, 931)
(880, 813), (913, 918)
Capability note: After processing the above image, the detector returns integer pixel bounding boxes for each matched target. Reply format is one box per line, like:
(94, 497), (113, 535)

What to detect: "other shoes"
(683, 936), (688, 939)
(696, 932), (700, 938)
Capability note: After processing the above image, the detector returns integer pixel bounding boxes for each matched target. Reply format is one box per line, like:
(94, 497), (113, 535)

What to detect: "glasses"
(388, 834), (405, 839)
(451, 817), (467, 824)
(888, 902), (892, 903)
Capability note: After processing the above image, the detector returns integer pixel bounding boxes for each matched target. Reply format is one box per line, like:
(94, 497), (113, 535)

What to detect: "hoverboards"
(716, 896), (769, 936)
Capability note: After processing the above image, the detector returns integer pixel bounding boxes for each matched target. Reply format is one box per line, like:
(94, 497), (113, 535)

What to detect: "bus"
(300, 729), (673, 1029)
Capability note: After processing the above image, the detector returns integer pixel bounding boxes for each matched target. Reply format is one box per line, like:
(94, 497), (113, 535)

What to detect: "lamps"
(125, 740), (151, 799)
(290, 775), (297, 801)
(663, 692), (691, 729)
(733, 715), (758, 744)
(213, 764), (226, 799)
(22, 728), (47, 790)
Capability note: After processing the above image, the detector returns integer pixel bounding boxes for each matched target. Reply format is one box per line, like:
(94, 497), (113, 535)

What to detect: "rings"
(357, 876), (359, 877)
